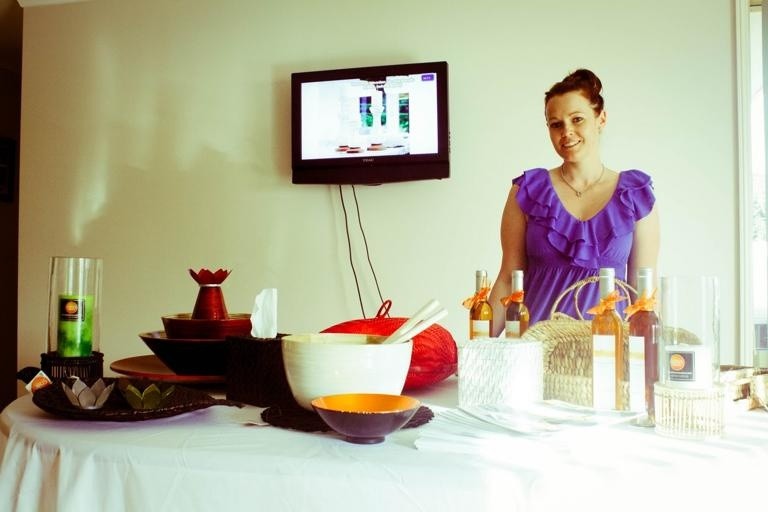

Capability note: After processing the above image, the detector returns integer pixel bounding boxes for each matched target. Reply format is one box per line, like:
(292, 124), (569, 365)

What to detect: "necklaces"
(559, 162), (604, 198)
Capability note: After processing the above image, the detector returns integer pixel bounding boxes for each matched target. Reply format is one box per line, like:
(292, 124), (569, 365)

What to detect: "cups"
(455, 337), (547, 421)
(48, 256), (100, 359)
(660, 273), (722, 395)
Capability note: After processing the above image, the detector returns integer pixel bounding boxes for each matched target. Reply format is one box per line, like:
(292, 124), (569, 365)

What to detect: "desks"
(0, 373), (768, 512)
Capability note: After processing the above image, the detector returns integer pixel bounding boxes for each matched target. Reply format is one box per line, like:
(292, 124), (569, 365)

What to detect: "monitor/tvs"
(291, 61), (450, 185)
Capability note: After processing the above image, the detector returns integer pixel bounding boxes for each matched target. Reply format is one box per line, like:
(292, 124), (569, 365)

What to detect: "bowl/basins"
(161, 314), (256, 338)
(310, 392), (424, 443)
(136, 330), (231, 375)
(282, 332), (415, 411)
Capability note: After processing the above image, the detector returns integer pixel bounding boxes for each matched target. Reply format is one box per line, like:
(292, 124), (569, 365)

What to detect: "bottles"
(188, 268), (232, 320)
(628, 268), (662, 428)
(590, 267), (624, 414)
(503, 269), (529, 338)
(470, 270), (494, 342)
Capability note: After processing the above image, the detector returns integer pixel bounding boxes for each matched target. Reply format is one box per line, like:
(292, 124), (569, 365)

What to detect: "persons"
(485, 68), (660, 338)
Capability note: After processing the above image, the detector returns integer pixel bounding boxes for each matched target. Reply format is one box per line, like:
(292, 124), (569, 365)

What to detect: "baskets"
(652, 380), (726, 437)
(522, 275), (701, 413)
